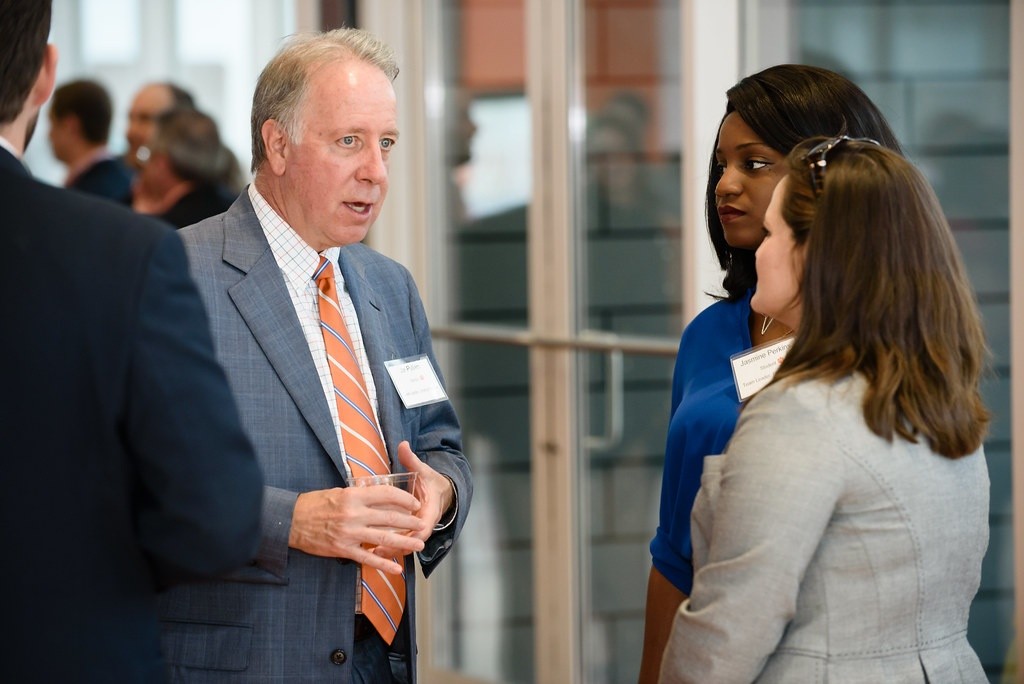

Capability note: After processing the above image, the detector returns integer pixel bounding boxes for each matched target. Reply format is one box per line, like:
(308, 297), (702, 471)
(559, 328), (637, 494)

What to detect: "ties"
(313, 255), (405, 646)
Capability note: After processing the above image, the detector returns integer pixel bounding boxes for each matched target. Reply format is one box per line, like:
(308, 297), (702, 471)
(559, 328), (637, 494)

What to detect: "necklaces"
(760, 316), (774, 335)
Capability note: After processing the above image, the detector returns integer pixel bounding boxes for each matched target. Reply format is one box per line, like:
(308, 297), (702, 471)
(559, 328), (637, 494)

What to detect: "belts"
(354, 614), (376, 642)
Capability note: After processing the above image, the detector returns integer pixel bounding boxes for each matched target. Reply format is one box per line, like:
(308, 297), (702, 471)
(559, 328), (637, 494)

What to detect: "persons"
(177, 26), (475, 684)
(635, 64), (906, 683)
(0, 0), (268, 684)
(137, 106), (244, 229)
(659, 135), (993, 683)
(121, 83), (193, 207)
(44, 79), (134, 205)
(584, 90), (684, 524)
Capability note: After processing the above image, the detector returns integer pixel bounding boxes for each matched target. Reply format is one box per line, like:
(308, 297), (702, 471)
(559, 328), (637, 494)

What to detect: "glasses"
(808, 135), (882, 211)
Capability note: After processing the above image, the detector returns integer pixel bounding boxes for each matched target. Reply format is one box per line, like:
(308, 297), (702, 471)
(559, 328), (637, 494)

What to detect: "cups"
(345, 471), (419, 536)
